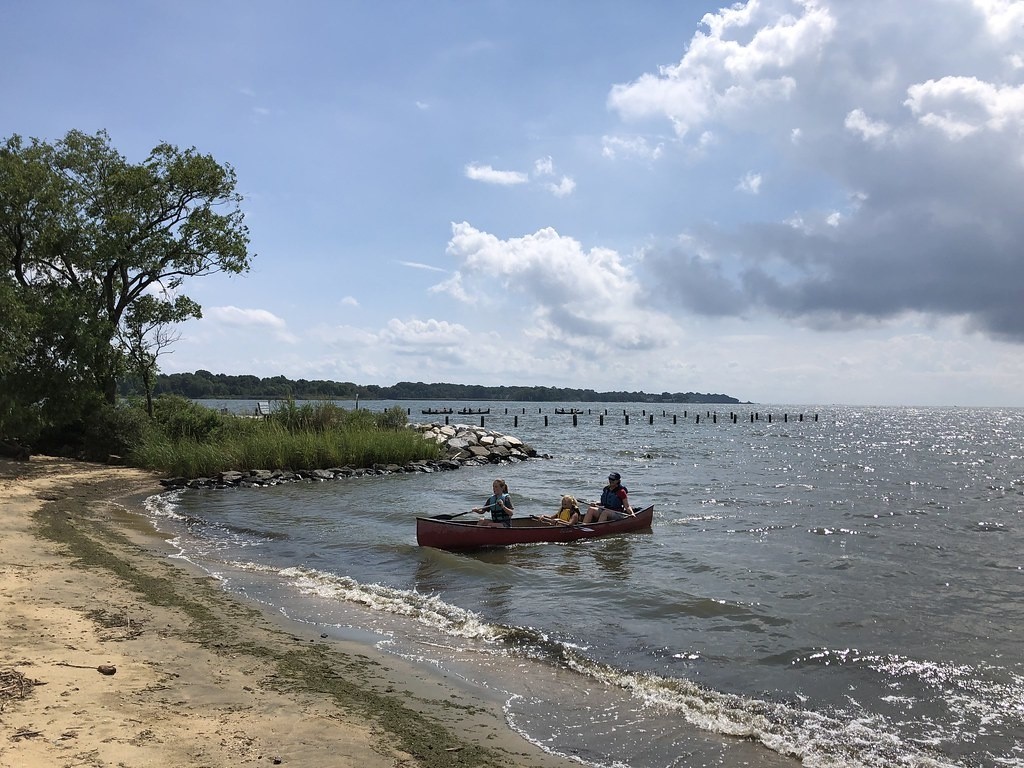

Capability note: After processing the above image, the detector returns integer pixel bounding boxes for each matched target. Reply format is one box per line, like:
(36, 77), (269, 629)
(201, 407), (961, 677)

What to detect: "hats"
(608, 472), (621, 479)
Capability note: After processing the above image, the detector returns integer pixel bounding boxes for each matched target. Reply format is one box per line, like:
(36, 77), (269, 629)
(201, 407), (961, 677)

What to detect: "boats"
(416, 502), (655, 550)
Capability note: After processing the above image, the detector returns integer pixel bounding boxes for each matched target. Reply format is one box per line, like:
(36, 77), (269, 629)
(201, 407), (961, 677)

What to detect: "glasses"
(609, 478), (615, 481)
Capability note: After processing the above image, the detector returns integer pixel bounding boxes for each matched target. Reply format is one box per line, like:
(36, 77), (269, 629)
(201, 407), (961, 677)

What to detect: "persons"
(538, 495), (581, 526)
(472, 479), (514, 527)
(582, 472), (636, 523)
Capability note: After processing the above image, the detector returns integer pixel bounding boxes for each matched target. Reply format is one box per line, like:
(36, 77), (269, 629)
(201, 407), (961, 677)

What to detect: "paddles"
(427, 503), (498, 521)
(560, 495), (631, 517)
